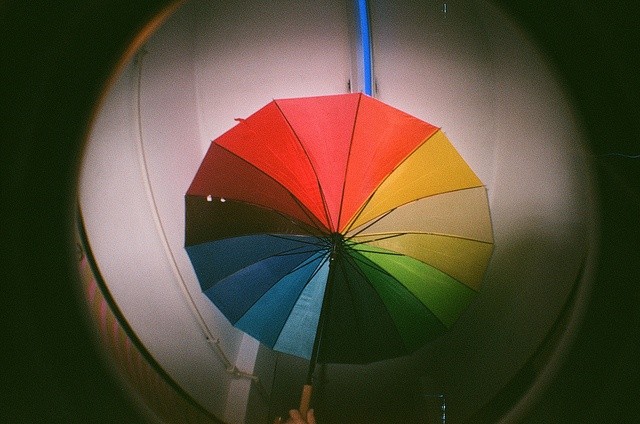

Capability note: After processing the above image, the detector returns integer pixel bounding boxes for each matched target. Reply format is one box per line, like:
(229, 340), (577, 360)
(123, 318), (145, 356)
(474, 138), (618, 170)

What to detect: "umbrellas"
(184, 90), (496, 421)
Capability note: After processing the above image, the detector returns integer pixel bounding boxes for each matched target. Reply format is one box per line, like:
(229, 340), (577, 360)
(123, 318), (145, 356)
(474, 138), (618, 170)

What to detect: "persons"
(285, 407), (316, 424)
